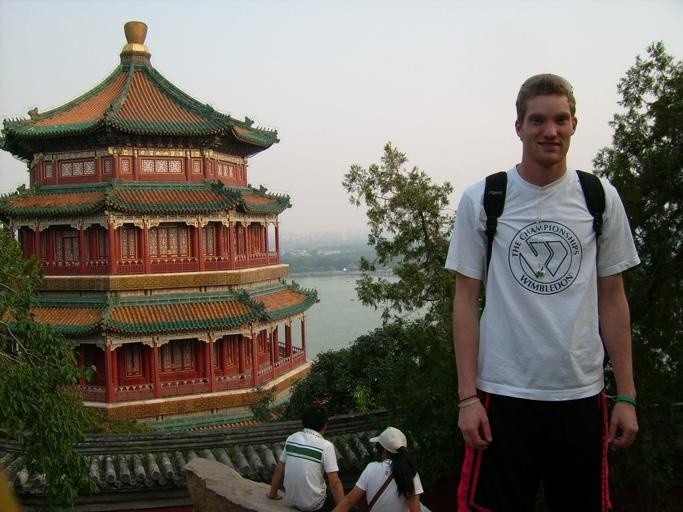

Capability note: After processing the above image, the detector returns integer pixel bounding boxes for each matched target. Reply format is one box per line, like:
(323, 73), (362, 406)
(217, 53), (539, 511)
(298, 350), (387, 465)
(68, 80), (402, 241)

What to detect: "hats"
(369, 427), (408, 454)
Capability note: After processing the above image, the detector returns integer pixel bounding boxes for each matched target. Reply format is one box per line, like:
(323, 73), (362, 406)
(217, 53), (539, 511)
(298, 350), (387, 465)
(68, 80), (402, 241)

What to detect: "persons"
(442, 72), (642, 512)
(330, 426), (425, 512)
(265, 404), (345, 512)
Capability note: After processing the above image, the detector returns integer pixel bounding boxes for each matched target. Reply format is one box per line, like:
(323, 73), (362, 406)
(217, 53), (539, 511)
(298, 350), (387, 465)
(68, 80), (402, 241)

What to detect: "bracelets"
(615, 395), (637, 406)
(457, 398), (480, 408)
(458, 394), (478, 403)
